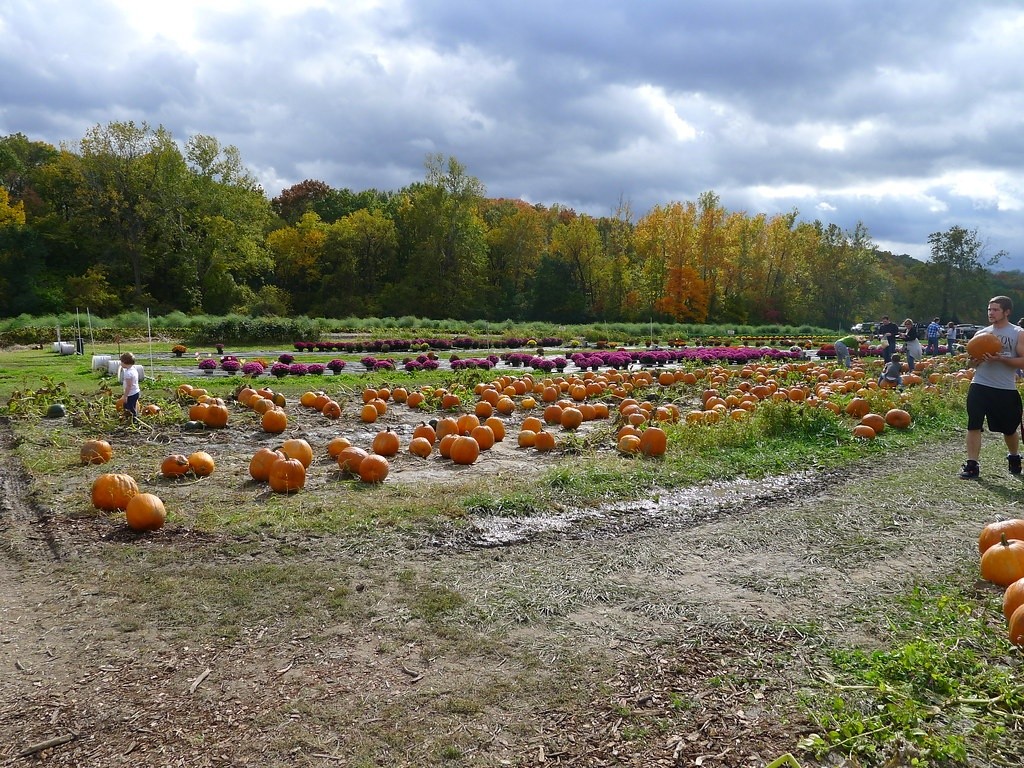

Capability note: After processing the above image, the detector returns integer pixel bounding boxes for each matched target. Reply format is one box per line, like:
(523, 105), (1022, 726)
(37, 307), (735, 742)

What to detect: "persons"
(898, 318), (916, 374)
(926, 317), (943, 357)
(945, 322), (956, 356)
(878, 354), (903, 392)
(960, 295), (1024, 479)
(1016, 318), (1024, 382)
(878, 315), (899, 363)
(119, 352), (139, 424)
(835, 335), (861, 368)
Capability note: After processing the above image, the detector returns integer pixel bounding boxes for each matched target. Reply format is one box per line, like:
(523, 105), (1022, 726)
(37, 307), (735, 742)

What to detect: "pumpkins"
(979, 515), (1024, 647)
(967, 332), (1003, 360)
(48, 353), (975, 532)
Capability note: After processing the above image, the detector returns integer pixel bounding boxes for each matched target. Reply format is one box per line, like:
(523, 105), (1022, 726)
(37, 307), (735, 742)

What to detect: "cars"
(957, 324), (977, 339)
(851, 324), (863, 332)
(975, 326), (981, 331)
(898, 323), (907, 332)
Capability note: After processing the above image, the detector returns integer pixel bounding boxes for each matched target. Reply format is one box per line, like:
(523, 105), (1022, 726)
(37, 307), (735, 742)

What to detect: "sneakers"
(959, 463), (979, 479)
(1006, 454), (1022, 474)
